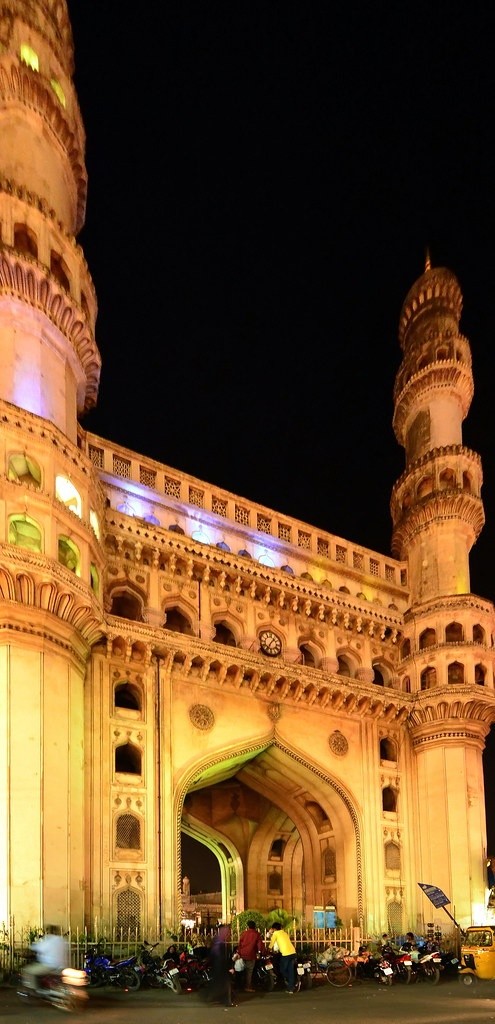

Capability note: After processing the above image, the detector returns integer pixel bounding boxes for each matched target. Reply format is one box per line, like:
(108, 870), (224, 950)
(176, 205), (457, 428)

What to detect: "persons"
(212, 921), (301, 1007)
(162, 946), (178, 960)
(23, 924), (68, 995)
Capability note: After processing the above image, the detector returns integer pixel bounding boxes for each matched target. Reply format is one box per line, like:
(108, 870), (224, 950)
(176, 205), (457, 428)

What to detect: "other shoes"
(284, 990), (294, 994)
(245, 988), (255, 993)
(228, 1003), (239, 1008)
(15, 988), (33, 997)
(295, 982), (301, 993)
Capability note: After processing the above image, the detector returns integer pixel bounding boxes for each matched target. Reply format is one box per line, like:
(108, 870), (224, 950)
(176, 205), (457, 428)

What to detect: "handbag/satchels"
(234, 958), (245, 972)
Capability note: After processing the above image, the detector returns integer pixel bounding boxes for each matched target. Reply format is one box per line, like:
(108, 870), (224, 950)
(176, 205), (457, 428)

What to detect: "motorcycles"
(163, 923), (460, 996)
(458, 925), (495, 987)
(135, 941), (182, 996)
(82, 938), (142, 992)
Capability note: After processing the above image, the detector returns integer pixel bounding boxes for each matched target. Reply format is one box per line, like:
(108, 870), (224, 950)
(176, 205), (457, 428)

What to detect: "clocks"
(259, 631), (283, 657)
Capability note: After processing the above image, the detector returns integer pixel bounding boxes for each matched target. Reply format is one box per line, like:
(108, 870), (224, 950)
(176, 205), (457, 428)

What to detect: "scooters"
(14, 946), (92, 1014)
